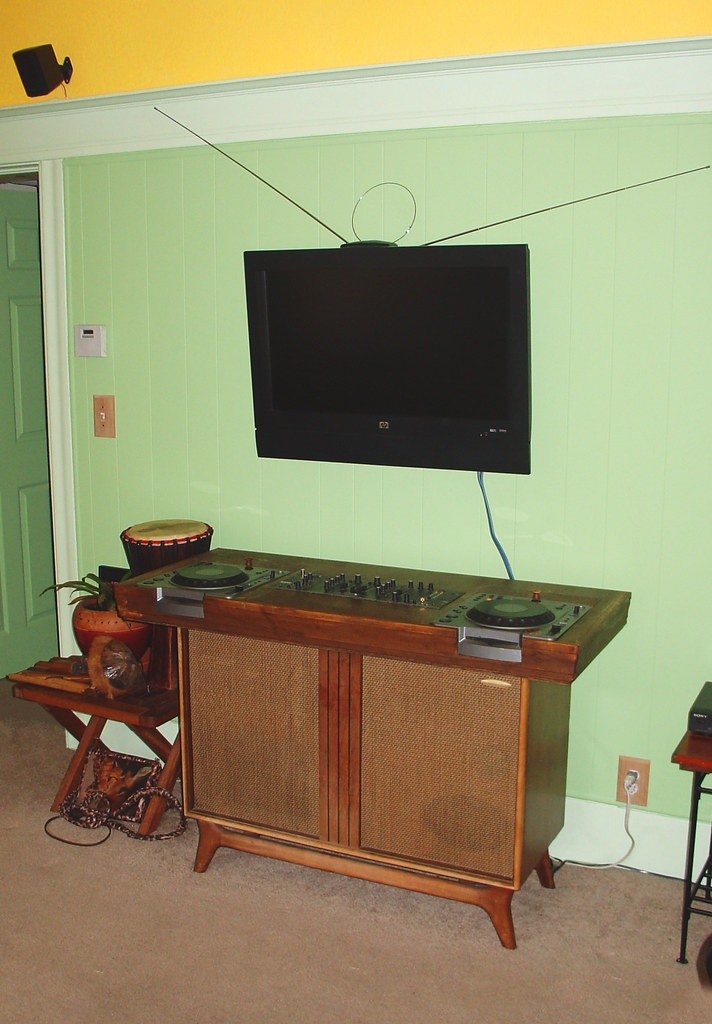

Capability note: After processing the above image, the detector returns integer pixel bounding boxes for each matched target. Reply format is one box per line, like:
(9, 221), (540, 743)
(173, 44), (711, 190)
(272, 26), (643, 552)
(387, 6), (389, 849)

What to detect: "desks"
(671, 732), (712, 964)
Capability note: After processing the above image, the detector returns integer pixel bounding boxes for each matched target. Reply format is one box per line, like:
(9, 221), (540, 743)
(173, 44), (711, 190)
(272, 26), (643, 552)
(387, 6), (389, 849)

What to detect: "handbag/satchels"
(58, 748), (188, 840)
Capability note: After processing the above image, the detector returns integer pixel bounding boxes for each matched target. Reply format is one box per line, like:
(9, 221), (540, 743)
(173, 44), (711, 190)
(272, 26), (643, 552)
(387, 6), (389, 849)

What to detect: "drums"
(120, 520), (214, 691)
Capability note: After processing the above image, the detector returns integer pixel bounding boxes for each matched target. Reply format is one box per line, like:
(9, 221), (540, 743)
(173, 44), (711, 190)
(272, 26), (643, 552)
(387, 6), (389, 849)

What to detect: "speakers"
(12, 43), (64, 97)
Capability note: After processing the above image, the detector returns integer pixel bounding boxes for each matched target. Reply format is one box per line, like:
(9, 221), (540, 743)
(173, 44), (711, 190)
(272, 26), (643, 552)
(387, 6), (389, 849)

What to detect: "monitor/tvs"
(243, 242), (533, 474)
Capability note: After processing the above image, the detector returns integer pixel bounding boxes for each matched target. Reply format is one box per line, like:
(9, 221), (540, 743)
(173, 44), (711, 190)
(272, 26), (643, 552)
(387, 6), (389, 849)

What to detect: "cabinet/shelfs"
(176, 626), (574, 950)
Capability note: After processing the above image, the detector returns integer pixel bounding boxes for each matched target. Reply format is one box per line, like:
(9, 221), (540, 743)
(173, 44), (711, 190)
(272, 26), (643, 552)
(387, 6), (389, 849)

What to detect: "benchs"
(11, 567), (185, 834)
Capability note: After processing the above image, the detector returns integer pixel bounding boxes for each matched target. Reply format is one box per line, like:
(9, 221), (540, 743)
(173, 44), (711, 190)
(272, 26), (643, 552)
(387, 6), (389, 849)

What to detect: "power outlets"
(616, 756), (650, 806)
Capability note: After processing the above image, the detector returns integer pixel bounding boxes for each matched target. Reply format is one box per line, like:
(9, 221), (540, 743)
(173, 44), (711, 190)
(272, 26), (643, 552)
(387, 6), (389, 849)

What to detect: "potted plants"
(41, 571), (149, 662)
